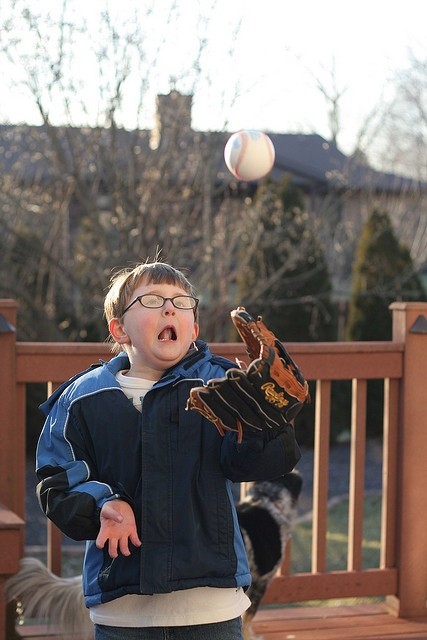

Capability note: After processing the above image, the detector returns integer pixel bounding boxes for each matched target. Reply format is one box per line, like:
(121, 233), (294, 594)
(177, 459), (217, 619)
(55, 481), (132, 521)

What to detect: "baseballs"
(224, 130), (275, 181)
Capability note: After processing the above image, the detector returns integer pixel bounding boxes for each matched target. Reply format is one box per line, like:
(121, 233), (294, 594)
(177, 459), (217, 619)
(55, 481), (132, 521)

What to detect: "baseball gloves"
(187, 307), (309, 442)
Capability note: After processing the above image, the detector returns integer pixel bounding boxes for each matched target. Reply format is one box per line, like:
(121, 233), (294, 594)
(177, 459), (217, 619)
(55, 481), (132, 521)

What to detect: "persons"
(34, 262), (302, 640)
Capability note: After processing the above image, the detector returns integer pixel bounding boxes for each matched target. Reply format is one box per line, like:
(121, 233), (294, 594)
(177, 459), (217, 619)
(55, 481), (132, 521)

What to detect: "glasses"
(120, 292), (199, 321)
